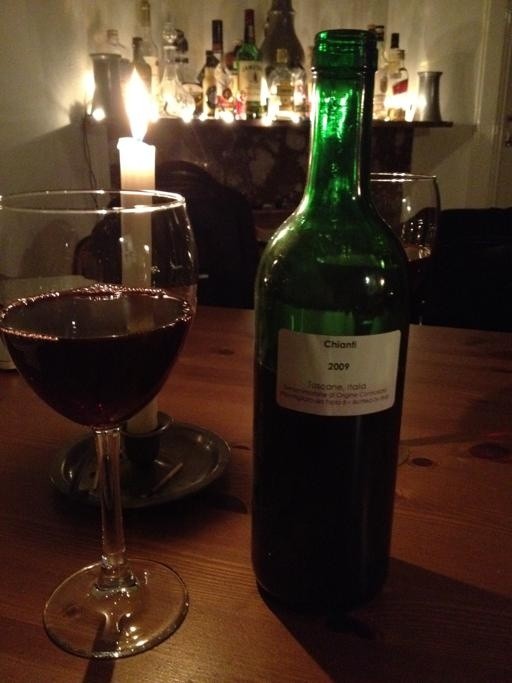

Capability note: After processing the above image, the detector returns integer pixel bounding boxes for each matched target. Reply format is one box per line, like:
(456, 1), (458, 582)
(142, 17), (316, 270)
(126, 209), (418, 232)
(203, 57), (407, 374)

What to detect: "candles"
(117, 66), (158, 433)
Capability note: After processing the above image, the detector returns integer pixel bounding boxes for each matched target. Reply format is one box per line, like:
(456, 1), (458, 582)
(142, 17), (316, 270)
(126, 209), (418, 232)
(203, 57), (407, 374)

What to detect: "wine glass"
(0, 188), (198, 661)
(364, 172), (443, 466)
(250, 28), (413, 620)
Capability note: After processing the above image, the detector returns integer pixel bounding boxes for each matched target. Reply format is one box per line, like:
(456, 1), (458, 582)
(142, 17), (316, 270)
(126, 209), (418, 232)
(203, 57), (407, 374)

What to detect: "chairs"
(396, 206), (511, 331)
(88, 163), (257, 310)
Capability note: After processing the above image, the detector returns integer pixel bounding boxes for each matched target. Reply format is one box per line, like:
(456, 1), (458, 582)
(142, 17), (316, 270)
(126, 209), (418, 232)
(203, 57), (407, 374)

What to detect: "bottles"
(101, 0), (236, 120)
(371, 26), (408, 121)
(267, 48), (296, 121)
(236, 8), (262, 120)
(256, 1), (310, 121)
